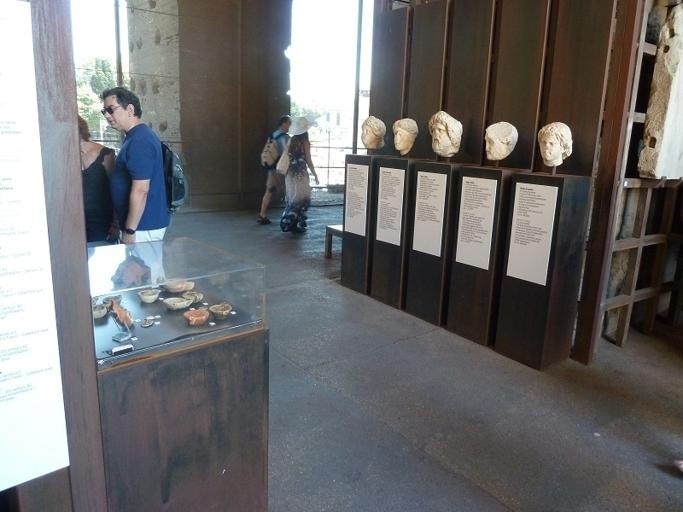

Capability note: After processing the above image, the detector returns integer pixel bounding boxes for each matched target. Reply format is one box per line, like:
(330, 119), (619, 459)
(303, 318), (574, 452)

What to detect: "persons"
(536, 121), (574, 168)
(111, 256), (151, 293)
(484, 119), (520, 162)
(76, 113), (122, 249)
(99, 85), (173, 287)
(427, 110), (464, 158)
(360, 114), (387, 150)
(392, 116), (420, 156)
(255, 114), (295, 225)
(279, 115), (320, 234)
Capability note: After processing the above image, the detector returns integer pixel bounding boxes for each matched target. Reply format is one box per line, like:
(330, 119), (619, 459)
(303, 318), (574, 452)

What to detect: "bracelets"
(123, 227), (137, 235)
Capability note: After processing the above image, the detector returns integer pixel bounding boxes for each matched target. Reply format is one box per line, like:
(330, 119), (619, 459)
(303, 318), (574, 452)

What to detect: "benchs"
(324, 224), (342, 259)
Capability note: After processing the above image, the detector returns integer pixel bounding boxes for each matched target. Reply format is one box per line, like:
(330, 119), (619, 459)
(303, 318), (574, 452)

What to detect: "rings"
(107, 232), (111, 234)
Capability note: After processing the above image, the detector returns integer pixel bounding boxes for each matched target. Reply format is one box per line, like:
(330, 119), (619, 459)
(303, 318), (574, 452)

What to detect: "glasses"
(101, 105), (122, 115)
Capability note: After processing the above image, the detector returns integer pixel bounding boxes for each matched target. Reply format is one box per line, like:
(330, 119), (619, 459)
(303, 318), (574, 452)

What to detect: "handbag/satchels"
(276, 142), (289, 175)
(162, 139), (188, 214)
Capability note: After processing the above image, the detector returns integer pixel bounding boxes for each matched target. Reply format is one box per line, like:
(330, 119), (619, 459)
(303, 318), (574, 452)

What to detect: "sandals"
(257, 215), (271, 224)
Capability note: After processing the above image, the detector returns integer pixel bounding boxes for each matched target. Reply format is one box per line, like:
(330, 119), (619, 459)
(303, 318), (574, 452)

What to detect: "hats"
(288, 118), (313, 136)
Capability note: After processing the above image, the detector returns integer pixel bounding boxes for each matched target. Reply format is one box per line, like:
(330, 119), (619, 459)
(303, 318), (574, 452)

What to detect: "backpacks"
(261, 133), (288, 170)
